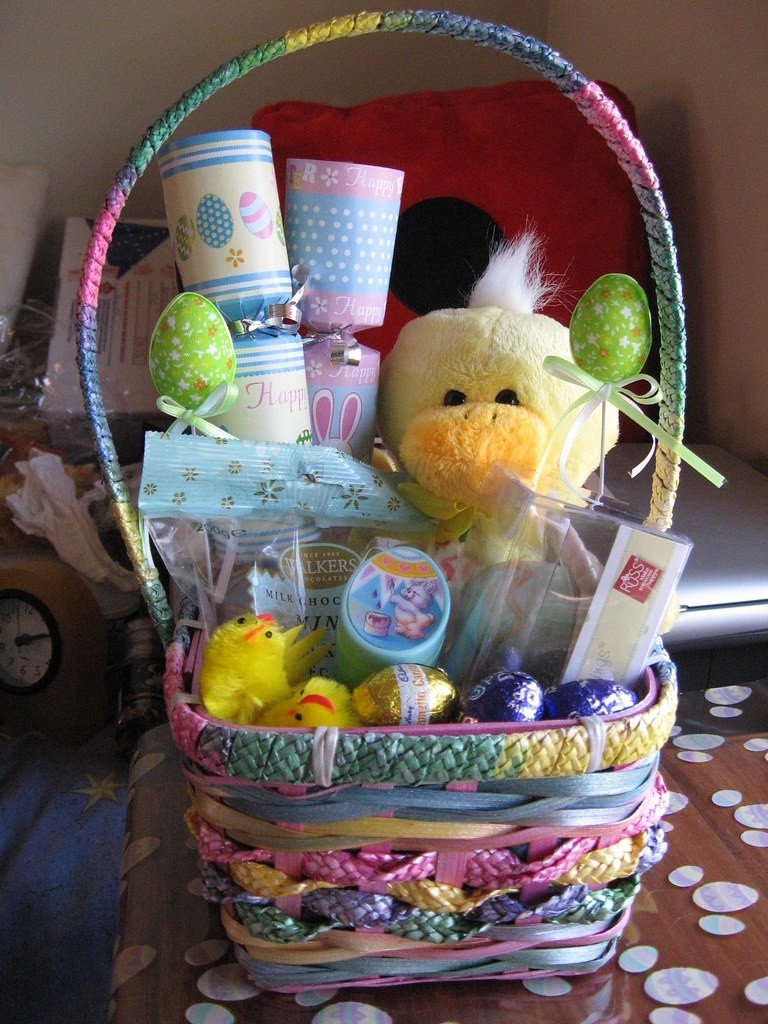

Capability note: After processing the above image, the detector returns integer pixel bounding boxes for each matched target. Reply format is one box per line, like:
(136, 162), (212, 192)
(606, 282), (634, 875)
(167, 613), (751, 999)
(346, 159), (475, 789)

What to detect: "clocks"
(0, 556), (121, 740)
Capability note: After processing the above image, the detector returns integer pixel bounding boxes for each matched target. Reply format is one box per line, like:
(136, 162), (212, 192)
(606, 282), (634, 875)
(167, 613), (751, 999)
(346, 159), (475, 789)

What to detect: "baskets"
(74, 10), (688, 994)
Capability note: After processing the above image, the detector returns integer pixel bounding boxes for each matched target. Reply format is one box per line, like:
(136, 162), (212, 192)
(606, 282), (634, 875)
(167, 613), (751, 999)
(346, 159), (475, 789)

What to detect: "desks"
(112, 714), (768, 1024)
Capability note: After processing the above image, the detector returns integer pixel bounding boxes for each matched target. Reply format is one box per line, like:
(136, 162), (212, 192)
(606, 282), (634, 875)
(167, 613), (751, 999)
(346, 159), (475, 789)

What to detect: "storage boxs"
(30, 216), (181, 463)
(438, 457), (698, 705)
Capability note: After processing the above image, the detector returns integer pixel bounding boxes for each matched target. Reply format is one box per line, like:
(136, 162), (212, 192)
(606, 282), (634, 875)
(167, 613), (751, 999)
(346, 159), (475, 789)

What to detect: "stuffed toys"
(197, 225), (619, 724)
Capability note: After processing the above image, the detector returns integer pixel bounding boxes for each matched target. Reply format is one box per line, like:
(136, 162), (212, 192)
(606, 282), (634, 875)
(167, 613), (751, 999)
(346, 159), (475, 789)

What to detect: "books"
(45, 215), (183, 414)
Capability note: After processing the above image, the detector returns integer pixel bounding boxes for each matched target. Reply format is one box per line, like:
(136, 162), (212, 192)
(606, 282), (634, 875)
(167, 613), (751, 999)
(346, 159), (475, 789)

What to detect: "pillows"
(250, 77), (654, 443)
